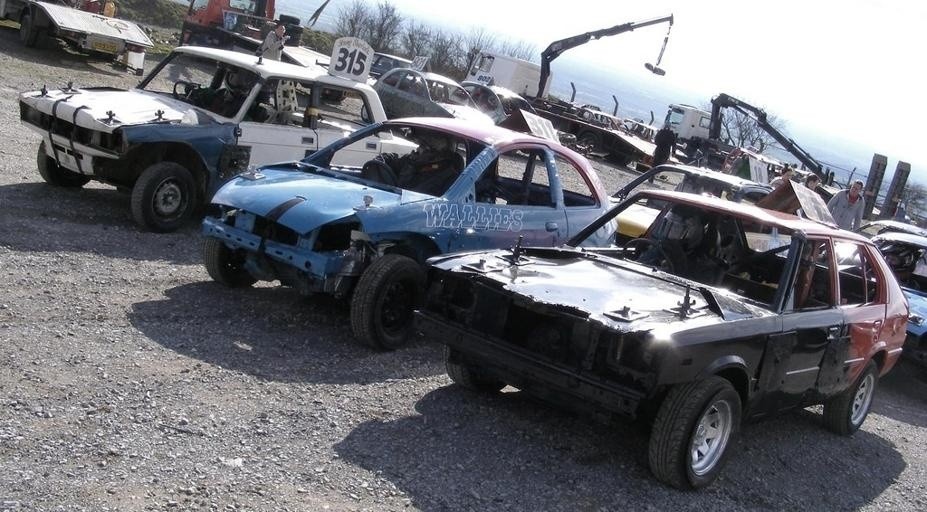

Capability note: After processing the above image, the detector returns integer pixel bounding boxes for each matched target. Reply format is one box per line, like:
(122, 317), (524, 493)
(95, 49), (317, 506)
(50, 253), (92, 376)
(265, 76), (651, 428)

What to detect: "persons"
(800, 173), (822, 192)
(770, 163), (793, 190)
(829, 180), (865, 233)
(649, 121), (674, 182)
(257, 23), (291, 62)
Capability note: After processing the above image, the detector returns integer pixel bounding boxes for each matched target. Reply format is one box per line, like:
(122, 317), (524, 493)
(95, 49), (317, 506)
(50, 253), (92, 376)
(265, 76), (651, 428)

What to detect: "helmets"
(414, 133), (449, 164)
(656, 204), (705, 250)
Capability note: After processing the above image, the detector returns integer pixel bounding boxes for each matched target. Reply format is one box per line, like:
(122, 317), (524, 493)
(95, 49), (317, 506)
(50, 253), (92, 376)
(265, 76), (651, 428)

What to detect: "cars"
(367, 49), (414, 79)
(201, 116), (617, 353)
(445, 78), (577, 150)
(19, 43), (419, 236)
(360, 68), (494, 132)
(408, 188), (912, 492)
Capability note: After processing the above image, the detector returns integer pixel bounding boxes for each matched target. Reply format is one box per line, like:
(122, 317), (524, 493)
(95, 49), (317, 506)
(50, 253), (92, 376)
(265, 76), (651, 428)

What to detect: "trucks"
(1, 0), (155, 58)
(181, 0), (378, 103)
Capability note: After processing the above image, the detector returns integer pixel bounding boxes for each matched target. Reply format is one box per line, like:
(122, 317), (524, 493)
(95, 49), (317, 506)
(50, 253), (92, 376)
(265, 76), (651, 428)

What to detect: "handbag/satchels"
(255, 46), (262, 56)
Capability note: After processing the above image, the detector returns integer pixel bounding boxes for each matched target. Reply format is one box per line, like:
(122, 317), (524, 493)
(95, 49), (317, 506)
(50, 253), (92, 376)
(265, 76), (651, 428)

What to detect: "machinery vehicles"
(663, 90), (912, 224)
(459, 13), (686, 176)
